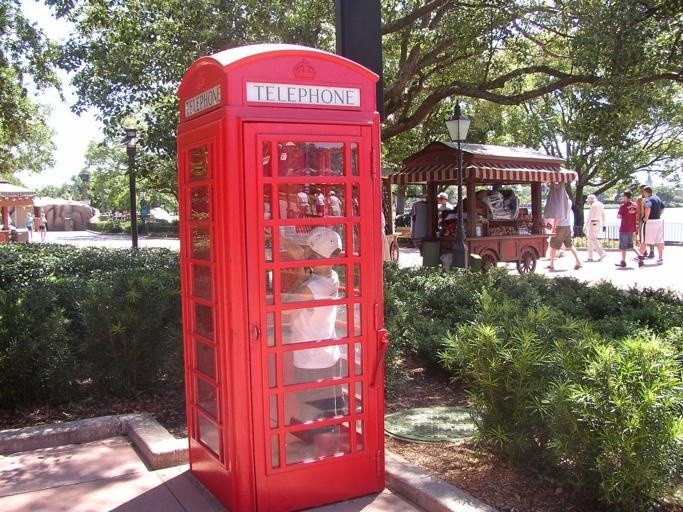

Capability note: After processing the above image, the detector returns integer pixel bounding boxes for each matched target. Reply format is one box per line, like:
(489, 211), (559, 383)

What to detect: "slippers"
(544, 266), (554, 270)
(574, 265), (583, 270)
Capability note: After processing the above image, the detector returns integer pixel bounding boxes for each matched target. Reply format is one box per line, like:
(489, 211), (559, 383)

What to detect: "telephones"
(303, 254), (318, 271)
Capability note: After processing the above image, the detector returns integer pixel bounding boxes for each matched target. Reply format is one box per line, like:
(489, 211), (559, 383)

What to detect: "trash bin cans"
(63, 217), (72, 231)
(11, 228), (28, 242)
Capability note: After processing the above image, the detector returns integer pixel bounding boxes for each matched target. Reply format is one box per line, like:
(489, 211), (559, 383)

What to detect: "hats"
(585, 194), (597, 202)
(293, 226), (343, 258)
(437, 192), (449, 200)
(329, 191), (335, 195)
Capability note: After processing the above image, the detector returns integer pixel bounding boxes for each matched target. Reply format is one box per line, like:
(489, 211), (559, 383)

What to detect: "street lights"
(123, 126), (138, 249)
(444, 101), (471, 268)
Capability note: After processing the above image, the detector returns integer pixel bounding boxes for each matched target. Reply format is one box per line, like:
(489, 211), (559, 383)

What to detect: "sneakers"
(583, 254), (606, 262)
(615, 251), (663, 268)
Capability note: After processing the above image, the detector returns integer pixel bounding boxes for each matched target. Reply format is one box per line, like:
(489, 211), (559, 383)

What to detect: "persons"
(39, 213), (47, 242)
(26, 213), (33, 238)
(642, 186), (665, 265)
(264, 226), (343, 445)
(140, 200), (151, 237)
(615, 190), (643, 266)
(545, 198), (582, 270)
(381, 211), (390, 261)
(313, 188), (325, 217)
(584, 194), (606, 262)
(437, 192), (454, 211)
(295, 188), (312, 214)
(638, 185), (654, 257)
(327, 190), (342, 216)
